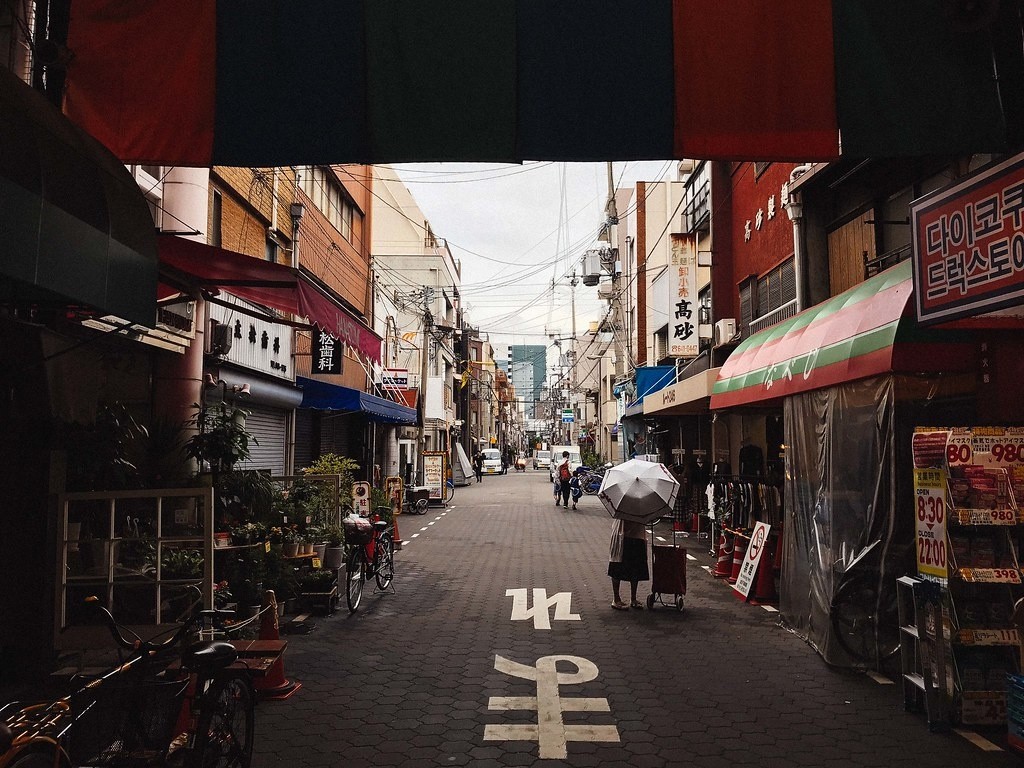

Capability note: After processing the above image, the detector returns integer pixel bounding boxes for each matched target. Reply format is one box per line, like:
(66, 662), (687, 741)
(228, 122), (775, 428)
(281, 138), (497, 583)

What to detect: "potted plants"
(68, 403), (359, 631)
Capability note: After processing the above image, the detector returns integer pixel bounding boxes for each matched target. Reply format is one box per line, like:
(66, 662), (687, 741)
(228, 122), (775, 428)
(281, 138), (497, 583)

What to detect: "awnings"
(0, 64), (157, 325)
(157, 234), (383, 367)
(626, 403), (643, 417)
(643, 367), (720, 414)
(296, 375), (417, 423)
(709, 257), (979, 409)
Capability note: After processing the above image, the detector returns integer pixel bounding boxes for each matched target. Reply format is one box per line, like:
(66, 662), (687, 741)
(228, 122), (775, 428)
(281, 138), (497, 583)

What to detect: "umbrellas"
(597, 459), (680, 524)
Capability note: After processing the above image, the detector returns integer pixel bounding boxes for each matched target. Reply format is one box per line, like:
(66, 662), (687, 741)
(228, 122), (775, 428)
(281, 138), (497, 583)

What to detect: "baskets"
(343, 522), (374, 544)
(70, 674), (184, 768)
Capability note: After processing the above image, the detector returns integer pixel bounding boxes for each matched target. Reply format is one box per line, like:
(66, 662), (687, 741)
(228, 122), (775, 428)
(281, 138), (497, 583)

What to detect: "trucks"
(549, 444), (585, 483)
(532, 450), (551, 470)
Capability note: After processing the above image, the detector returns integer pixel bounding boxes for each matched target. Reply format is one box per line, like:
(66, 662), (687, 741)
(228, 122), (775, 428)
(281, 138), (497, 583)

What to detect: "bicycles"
(0, 584), (258, 768)
(400, 470), (455, 505)
(577, 464), (615, 495)
(339, 501), (396, 612)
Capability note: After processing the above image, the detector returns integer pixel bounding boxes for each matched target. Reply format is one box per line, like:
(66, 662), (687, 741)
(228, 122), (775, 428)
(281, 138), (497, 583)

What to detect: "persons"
(607, 524), (649, 610)
(502, 455), (508, 474)
(553, 451), (582, 510)
(475, 451), (484, 483)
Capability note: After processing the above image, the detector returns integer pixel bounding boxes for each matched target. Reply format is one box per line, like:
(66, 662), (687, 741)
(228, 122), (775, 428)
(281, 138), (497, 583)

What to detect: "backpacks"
(570, 478), (582, 498)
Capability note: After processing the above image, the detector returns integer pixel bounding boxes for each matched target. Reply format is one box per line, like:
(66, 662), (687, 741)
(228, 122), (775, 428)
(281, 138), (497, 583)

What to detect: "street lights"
(586, 354), (602, 465)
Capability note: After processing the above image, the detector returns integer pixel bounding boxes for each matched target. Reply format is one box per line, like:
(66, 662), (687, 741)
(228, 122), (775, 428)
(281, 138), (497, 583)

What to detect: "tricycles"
(380, 476), (431, 515)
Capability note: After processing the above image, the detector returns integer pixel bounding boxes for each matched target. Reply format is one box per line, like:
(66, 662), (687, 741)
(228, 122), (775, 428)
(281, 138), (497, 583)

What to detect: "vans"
(476, 448), (502, 475)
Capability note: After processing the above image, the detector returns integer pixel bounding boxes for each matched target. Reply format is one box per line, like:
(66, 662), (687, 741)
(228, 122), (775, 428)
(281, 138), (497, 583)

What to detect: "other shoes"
(563, 503), (568, 509)
(556, 503), (560, 506)
(572, 506), (577, 510)
(476, 479), (479, 483)
(480, 480), (481, 482)
(631, 600), (644, 608)
(611, 600), (629, 610)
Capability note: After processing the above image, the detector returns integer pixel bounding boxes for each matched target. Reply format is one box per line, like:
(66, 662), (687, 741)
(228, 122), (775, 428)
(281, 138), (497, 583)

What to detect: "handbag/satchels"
(622, 537), (647, 560)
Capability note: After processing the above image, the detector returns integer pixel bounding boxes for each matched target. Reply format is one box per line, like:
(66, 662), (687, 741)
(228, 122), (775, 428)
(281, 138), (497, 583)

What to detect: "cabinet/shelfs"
(912, 431), (1024, 725)
